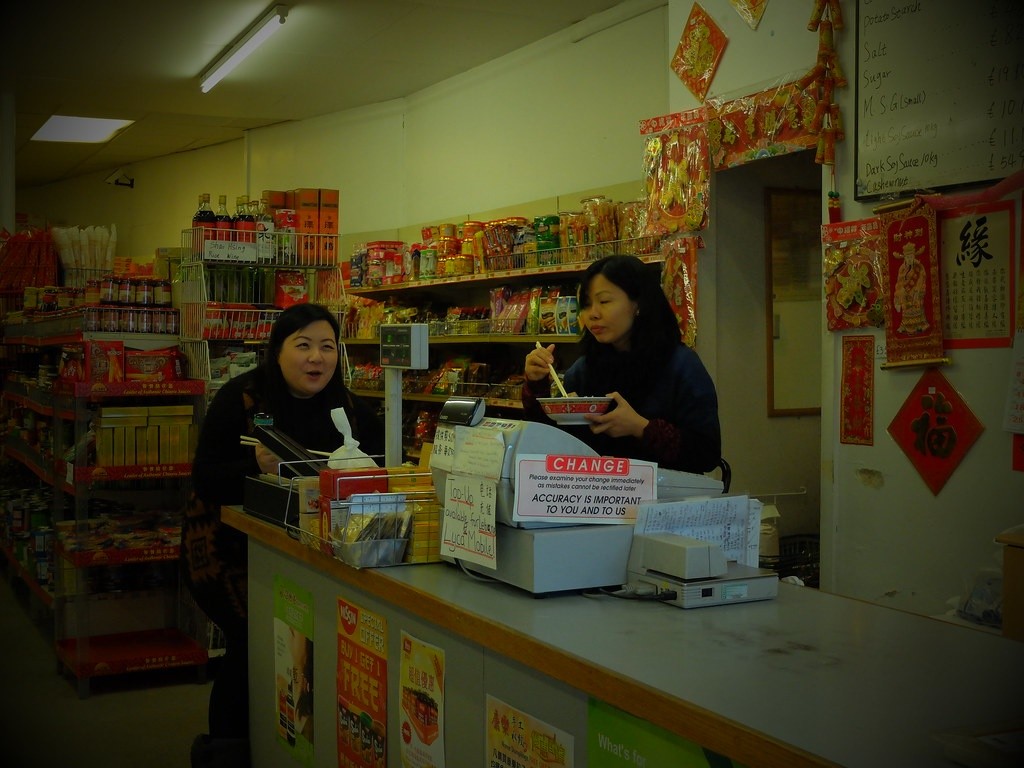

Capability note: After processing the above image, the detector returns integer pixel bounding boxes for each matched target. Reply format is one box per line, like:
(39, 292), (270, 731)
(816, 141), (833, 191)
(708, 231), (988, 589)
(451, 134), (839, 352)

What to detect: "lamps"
(196, 3), (287, 94)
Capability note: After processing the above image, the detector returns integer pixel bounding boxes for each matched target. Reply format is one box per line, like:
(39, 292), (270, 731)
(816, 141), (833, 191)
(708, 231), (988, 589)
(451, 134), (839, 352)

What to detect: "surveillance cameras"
(103, 169), (124, 184)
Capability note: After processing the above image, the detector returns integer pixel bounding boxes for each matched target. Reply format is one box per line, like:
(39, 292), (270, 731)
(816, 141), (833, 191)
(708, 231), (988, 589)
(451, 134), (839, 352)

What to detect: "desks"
(221, 505), (1024, 768)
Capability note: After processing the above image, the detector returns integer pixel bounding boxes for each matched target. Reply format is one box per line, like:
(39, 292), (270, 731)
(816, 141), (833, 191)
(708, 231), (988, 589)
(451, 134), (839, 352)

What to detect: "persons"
(182, 303), (384, 768)
(520, 254), (722, 475)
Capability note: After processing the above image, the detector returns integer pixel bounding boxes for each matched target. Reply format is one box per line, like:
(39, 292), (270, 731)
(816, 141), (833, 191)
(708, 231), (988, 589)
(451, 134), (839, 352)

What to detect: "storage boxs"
(328, 531), (408, 570)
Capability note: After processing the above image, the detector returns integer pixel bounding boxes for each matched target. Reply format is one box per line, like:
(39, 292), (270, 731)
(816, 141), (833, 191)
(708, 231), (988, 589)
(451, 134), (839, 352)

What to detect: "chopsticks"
(240, 434), (334, 456)
(536, 341), (569, 398)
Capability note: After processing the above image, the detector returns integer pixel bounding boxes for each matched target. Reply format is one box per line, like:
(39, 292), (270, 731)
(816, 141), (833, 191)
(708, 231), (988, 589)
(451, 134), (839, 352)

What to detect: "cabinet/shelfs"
(343, 236), (666, 458)
(1, 227), (341, 700)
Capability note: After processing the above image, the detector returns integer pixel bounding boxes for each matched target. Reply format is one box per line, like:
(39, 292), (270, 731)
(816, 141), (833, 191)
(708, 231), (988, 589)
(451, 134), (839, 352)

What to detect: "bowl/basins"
(537, 397), (612, 425)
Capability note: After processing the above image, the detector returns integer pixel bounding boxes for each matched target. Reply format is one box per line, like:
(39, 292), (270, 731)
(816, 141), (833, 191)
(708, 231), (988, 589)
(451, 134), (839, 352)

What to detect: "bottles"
(193, 194), (295, 265)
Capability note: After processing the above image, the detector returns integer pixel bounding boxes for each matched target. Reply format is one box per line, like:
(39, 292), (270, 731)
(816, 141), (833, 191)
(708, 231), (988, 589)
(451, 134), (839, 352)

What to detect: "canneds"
(24, 257), (180, 336)
(0, 347), (81, 590)
